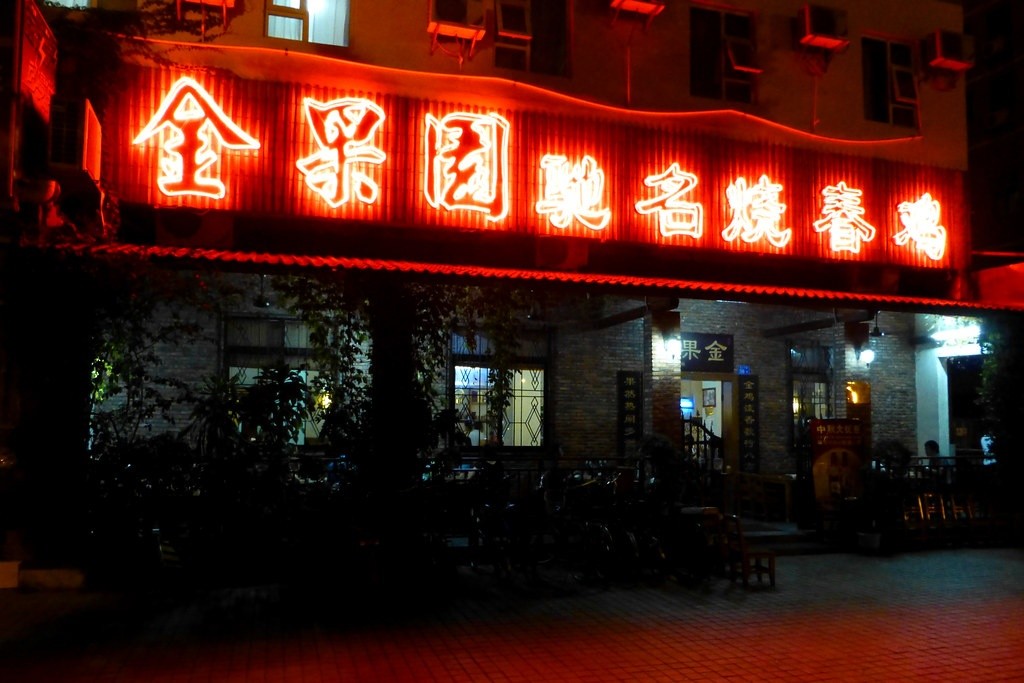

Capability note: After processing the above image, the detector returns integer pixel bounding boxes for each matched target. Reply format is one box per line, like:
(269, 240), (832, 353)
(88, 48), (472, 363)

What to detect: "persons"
(467, 421), (485, 446)
(914, 440), (950, 478)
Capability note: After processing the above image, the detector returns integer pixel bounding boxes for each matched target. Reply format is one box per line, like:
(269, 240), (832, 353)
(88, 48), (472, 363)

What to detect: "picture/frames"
(702, 388), (715, 408)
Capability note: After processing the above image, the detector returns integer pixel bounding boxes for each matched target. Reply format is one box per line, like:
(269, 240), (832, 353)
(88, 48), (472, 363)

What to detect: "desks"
(454, 468), (530, 492)
(578, 466), (639, 515)
(904, 470), (930, 511)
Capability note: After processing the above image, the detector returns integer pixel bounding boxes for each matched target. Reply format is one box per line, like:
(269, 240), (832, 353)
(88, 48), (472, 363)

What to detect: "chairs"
(727, 520), (782, 590)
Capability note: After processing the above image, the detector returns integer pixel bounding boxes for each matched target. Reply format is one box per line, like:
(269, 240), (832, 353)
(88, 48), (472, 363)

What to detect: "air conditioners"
(926, 28), (976, 71)
(798, 2), (850, 50)
(610, 0), (665, 17)
(428, 0), (487, 41)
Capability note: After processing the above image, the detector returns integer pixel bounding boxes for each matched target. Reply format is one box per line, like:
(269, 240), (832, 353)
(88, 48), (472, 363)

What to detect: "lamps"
(252, 272), (271, 309)
(869, 309), (884, 338)
